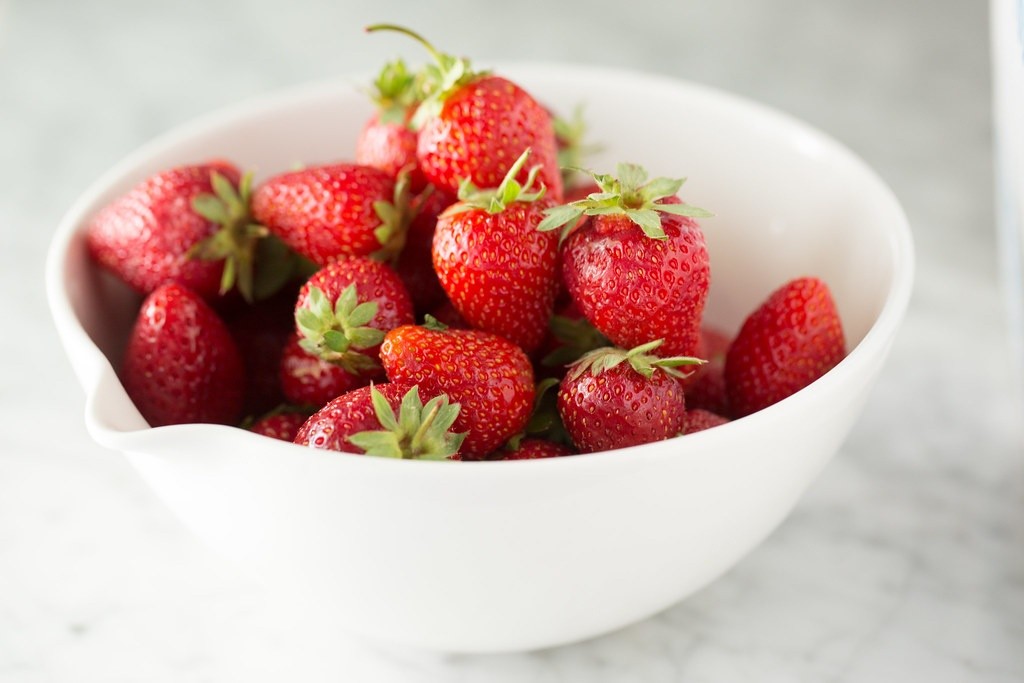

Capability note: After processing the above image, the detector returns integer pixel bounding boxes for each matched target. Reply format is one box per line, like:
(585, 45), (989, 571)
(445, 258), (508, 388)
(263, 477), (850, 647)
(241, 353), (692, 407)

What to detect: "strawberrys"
(85, 23), (847, 463)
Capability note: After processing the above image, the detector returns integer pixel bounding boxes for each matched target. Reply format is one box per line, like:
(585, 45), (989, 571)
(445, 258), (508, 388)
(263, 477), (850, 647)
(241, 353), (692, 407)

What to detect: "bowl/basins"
(44, 60), (916, 655)
